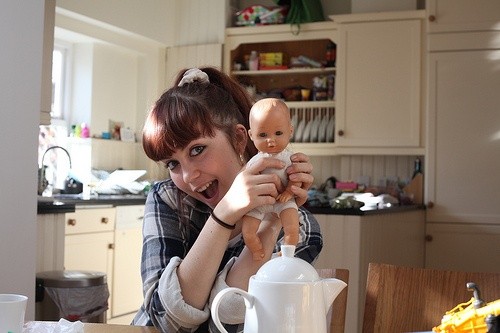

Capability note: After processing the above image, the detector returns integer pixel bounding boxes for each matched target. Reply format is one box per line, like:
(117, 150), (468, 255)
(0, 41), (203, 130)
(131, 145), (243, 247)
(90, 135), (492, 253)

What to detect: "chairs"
(362, 262), (500, 333)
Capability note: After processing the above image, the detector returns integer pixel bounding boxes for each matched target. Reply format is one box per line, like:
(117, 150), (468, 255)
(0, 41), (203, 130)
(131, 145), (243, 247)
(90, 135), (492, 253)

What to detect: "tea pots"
(210, 245), (348, 333)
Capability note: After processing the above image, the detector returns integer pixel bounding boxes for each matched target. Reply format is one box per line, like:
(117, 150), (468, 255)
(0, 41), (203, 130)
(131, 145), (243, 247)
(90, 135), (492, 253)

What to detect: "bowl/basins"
(282, 89), (300, 101)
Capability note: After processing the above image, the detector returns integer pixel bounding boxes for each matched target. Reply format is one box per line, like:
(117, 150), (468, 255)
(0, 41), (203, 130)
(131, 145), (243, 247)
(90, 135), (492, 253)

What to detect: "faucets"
(39, 146), (72, 196)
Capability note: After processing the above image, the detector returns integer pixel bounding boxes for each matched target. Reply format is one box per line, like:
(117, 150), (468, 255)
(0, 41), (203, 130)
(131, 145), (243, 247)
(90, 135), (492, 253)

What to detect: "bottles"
(248, 50), (258, 71)
(412, 160), (422, 180)
(70, 122), (90, 137)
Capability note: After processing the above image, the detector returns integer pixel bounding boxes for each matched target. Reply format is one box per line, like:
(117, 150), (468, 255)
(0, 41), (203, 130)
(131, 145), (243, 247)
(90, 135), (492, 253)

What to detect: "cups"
(103, 133), (110, 139)
(301, 89), (310, 100)
(0, 293), (28, 333)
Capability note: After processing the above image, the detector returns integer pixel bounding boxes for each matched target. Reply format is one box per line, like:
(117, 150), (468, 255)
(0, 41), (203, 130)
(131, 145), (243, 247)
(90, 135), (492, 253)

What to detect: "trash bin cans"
(36, 270), (110, 324)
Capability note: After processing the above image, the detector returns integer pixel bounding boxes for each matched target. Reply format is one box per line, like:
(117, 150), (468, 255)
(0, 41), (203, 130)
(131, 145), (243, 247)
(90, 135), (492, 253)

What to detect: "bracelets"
(209, 210), (236, 230)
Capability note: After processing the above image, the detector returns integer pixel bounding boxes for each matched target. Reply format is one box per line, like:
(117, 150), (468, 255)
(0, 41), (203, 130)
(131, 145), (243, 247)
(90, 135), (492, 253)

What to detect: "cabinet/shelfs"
(217, 0), (500, 333)
(36, 206), (146, 325)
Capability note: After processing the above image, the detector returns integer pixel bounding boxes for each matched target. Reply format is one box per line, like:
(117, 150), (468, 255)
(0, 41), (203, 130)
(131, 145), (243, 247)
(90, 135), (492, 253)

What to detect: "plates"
(37, 195), (54, 203)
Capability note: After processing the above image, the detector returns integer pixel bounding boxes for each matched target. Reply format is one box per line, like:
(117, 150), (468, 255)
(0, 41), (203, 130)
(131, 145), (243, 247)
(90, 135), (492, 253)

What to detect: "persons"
(242, 97), (302, 259)
(129, 67), (324, 333)
